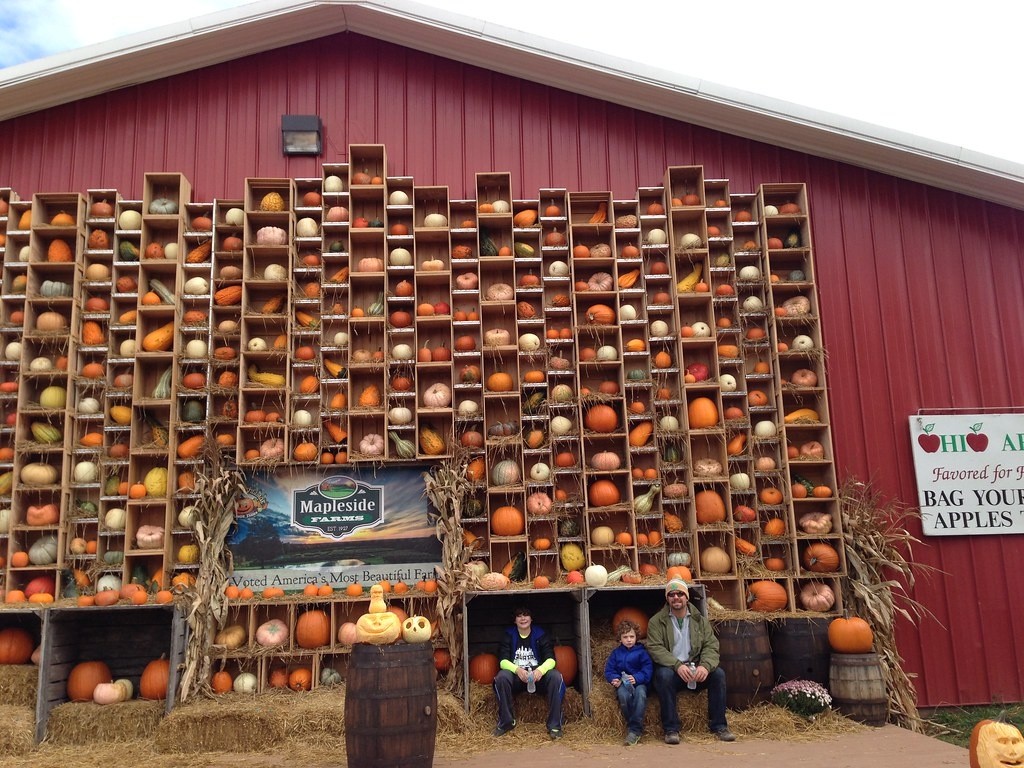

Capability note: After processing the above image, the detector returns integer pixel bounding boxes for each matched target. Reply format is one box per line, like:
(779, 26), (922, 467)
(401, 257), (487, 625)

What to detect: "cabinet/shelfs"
(1, 162), (849, 695)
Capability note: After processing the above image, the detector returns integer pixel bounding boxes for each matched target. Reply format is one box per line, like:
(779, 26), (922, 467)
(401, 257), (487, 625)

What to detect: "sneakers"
(550, 728), (562, 738)
(493, 719), (516, 736)
(665, 730), (679, 744)
(717, 728), (736, 741)
(624, 732), (641, 746)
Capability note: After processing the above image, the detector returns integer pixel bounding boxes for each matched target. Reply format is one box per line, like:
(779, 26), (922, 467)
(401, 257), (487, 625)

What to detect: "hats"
(665, 578), (689, 600)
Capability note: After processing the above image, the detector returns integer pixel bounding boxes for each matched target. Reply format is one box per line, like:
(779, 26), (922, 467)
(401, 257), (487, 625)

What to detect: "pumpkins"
(0, 168), (876, 705)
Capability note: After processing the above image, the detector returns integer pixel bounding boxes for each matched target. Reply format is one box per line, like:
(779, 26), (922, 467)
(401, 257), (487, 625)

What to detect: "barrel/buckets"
(344, 640), (437, 768)
(771, 618), (834, 690)
(714, 620), (774, 704)
(829, 653), (887, 727)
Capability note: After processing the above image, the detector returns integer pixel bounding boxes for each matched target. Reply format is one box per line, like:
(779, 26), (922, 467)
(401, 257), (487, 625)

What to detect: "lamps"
(280, 114), (322, 157)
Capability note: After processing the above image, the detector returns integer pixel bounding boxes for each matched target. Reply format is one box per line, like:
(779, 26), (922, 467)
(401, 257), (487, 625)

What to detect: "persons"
(494, 608), (566, 741)
(605, 620), (653, 747)
(647, 574), (735, 745)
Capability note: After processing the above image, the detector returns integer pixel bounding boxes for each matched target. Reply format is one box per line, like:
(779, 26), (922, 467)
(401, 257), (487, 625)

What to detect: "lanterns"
(354, 584), (431, 645)
(968, 709), (1024, 767)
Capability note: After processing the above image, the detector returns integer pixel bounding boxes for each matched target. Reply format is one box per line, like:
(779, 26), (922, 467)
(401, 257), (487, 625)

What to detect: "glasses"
(668, 592), (684, 597)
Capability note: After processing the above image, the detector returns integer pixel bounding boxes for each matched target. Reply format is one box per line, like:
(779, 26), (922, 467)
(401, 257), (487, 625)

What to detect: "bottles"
(526, 667), (536, 693)
(687, 662), (697, 689)
(621, 672), (636, 695)
